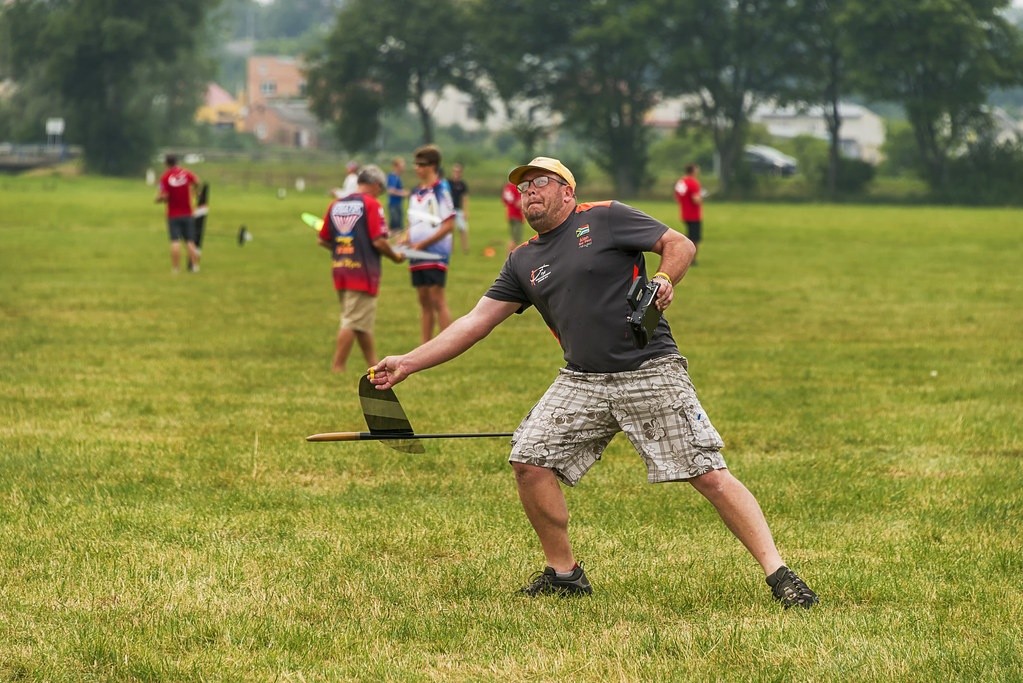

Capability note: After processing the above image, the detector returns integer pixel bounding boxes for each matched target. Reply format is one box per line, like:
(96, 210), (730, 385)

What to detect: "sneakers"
(764, 565), (819, 610)
(515, 561), (593, 598)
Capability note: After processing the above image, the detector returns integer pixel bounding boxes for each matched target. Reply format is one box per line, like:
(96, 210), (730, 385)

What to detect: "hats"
(509, 157), (576, 193)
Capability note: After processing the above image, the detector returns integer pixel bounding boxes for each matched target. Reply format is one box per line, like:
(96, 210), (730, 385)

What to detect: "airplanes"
(304, 373), (515, 455)
(300, 211), (448, 260)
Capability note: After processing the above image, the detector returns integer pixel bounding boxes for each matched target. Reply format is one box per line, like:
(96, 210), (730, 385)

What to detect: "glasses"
(516, 175), (567, 193)
(416, 163), (433, 168)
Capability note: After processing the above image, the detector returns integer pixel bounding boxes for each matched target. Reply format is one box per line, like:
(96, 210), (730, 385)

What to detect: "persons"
(675, 162), (705, 267)
(503, 181), (526, 250)
(315, 165), (406, 373)
(447, 162), (469, 254)
(383, 155), (412, 231)
(368, 156), (818, 610)
(155, 154), (202, 276)
(332, 160), (360, 198)
(400, 144), (457, 342)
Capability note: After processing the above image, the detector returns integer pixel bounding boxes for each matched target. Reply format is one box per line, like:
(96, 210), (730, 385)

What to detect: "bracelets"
(653, 272), (672, 285)
